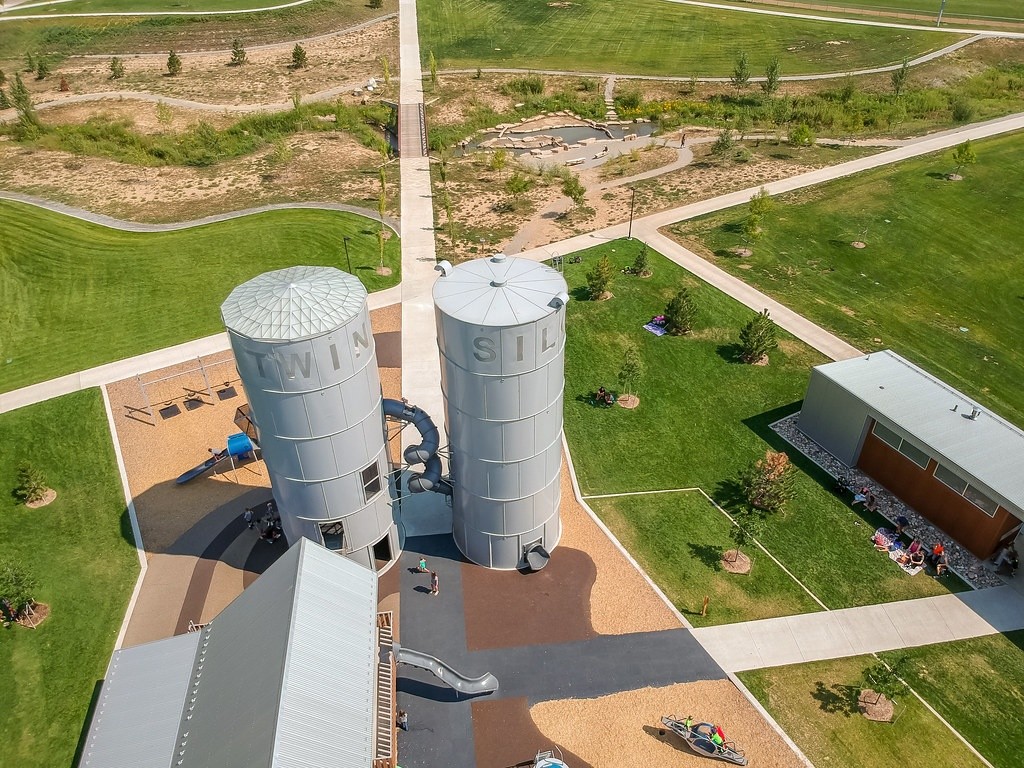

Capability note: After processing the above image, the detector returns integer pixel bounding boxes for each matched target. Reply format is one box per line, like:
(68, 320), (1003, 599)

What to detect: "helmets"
(689, 716), (693, 720)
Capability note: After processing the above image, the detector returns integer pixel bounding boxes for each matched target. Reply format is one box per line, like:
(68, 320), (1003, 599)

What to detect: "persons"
(417, 556), (430, 574)
(397, 710), (409, 731)
(851, 482), (949, 579)
(685, 716), (727, 754)
(430, 571), (439, 595)
(244, 502), (281, 543)
(208, 448), (224, 461)
(681, 134), (686, 149)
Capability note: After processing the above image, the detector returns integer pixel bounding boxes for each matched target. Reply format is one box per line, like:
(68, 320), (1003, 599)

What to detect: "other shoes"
(434, 591), (439, 595)
(430, 590), (434, 594)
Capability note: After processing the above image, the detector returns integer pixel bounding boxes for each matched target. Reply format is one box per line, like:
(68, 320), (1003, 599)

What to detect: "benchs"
(565, 158), (586, 167)
(623, 134), (637, 143)
(594, 150), (610, 160)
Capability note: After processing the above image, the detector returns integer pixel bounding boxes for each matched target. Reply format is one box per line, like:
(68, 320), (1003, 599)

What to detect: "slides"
(175, 448), (229, 485)
(527, 543), (551, 571)
(396, 648), (499, 696)
